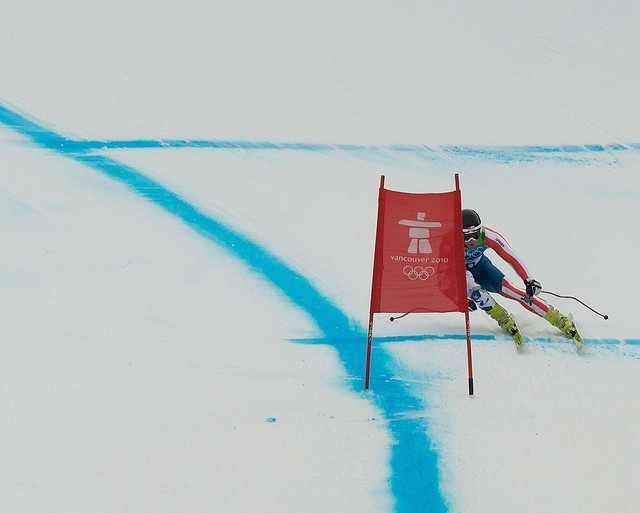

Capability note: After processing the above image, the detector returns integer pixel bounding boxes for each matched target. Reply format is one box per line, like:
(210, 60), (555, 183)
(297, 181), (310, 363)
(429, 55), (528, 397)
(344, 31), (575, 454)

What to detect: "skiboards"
(508, 312), (585, 354)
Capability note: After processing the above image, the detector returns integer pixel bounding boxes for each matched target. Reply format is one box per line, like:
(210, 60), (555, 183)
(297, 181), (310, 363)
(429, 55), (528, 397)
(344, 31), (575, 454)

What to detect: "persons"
(437, 209), (572, 333)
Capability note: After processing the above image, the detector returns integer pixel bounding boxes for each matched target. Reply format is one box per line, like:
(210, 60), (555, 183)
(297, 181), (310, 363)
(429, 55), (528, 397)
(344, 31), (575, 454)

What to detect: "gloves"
(524, 275), (542, 295)
(458, 296), (477, 312)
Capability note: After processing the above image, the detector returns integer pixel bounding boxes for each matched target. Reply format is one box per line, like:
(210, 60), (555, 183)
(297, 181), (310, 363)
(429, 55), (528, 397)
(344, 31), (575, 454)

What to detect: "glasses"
(464, 230), (480, 242)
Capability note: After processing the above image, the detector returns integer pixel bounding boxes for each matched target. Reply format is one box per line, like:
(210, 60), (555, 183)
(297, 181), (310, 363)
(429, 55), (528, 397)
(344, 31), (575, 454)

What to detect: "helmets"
(462, 208), (482, 232)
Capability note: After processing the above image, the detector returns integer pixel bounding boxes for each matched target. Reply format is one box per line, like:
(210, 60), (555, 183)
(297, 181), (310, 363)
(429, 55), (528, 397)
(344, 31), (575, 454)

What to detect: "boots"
(542, 305), (576, 336)
(486, 302), (518, 333)
